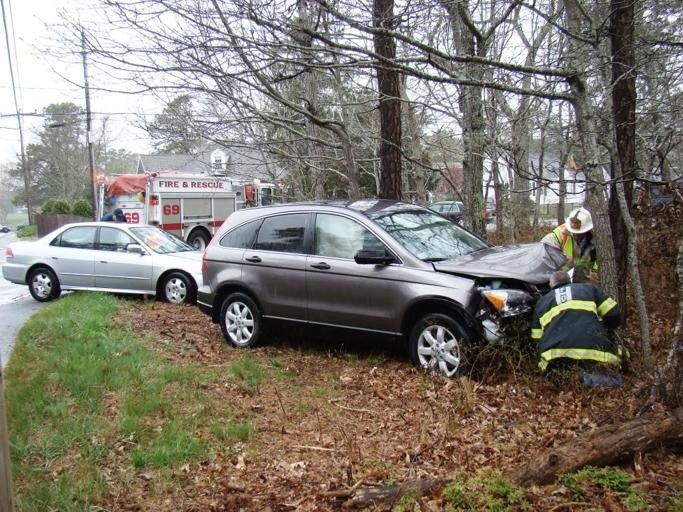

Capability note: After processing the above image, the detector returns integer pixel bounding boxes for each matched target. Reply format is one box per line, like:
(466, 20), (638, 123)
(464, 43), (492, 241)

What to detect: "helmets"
(566, 207), (594, 234)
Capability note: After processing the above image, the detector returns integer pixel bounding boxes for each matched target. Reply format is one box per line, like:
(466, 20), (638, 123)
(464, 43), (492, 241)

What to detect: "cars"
(1, 220), (205, 306)
(425, 199), (496, 227)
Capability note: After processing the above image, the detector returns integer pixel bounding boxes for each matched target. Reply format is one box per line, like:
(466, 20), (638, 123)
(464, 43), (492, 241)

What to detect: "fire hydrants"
(111, 171), (285, 253)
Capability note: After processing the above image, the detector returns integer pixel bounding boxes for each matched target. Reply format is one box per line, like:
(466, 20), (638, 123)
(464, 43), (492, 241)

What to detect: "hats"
(114, 208), (123, 217)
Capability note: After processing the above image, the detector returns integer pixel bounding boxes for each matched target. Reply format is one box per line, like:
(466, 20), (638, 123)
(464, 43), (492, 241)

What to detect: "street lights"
(48, 117), (99, 223)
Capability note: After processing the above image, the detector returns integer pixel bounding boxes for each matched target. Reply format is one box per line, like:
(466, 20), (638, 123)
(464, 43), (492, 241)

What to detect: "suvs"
(631, 183), (683, 229)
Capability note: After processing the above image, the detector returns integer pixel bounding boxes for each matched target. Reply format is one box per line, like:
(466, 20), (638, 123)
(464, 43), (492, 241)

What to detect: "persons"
(102, 208), (126, 222)
(540, 207), (598, 286)
(532, 271), (624, 387)
